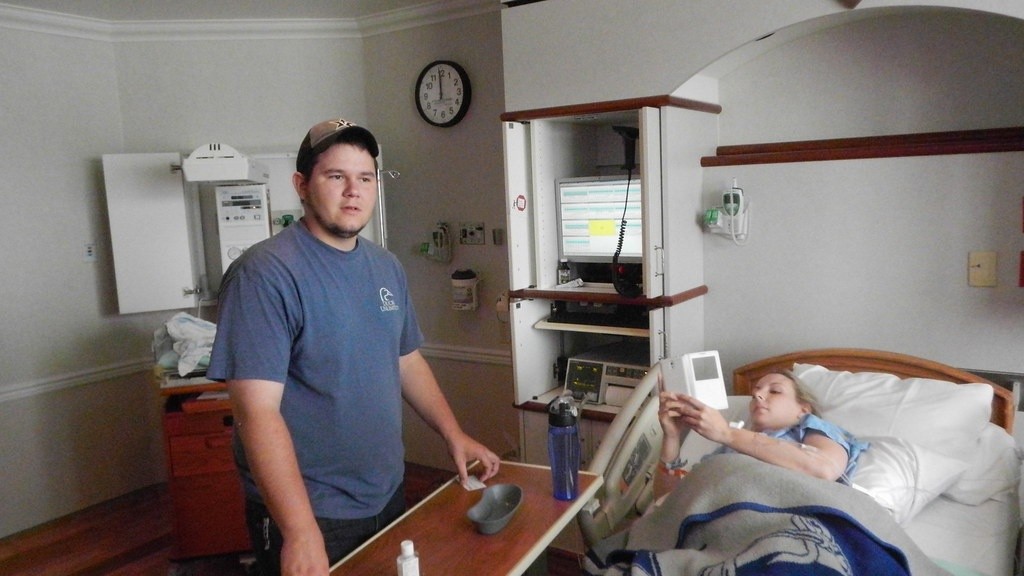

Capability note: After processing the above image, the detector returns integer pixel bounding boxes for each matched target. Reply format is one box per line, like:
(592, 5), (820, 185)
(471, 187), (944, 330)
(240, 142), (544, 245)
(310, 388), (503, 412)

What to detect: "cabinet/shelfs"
(500, 94), (723, 472)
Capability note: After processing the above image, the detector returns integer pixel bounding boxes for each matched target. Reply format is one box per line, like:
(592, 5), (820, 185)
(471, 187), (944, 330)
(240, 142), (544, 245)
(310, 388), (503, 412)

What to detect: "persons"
(652, 367), (871, 576)
(206, 116), (500, 575)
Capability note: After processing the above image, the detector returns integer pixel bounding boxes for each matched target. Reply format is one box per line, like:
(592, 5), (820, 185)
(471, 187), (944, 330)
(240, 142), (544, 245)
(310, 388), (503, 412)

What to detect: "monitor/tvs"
(554, 174), (644, 262)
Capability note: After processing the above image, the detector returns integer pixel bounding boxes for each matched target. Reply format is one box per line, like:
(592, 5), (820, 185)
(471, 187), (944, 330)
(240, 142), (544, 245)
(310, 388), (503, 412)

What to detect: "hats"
(296, 119), (380, 172)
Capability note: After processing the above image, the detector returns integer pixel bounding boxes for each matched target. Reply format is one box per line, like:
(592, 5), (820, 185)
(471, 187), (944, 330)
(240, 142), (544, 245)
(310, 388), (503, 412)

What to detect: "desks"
(328, 457), (608, 575)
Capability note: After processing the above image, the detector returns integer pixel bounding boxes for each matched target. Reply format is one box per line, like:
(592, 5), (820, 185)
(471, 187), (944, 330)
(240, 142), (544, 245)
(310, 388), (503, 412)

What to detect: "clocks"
(414, 59), (472, 129)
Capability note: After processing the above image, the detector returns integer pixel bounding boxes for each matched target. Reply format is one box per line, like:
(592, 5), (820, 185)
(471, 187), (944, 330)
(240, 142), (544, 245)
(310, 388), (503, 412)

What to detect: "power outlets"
(967, 251), (998, 288)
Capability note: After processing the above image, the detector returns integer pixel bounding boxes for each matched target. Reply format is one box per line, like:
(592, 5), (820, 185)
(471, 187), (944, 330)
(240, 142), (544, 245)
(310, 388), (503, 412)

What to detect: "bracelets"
(656, 455), (691, 478)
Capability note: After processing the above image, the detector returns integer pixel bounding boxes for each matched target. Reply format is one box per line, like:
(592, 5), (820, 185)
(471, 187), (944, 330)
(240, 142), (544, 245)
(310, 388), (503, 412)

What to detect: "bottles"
(547, 396), (580, 501)
(396, 539), (420, 575)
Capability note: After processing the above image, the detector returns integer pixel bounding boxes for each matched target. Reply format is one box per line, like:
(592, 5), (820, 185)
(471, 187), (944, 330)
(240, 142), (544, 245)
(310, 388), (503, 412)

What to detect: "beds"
(578, 346), (1024, 576)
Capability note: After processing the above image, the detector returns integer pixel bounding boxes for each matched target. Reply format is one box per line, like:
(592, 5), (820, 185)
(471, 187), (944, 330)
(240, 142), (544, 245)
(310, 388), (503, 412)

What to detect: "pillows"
(944, 422), (1015, 507)
(794, 361), (995, 524)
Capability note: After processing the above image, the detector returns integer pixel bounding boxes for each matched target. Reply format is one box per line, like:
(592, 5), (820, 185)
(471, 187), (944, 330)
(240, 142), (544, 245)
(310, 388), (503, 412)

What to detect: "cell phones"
(659, 356), (695, 417)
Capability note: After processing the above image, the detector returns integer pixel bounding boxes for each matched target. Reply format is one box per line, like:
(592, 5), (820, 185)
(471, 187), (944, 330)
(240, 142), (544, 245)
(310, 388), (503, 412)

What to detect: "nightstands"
(160, 372), (253, 560)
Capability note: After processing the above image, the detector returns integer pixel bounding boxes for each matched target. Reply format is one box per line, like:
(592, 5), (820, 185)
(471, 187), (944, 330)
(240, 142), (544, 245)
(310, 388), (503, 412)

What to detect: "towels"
(156, 311), (221, 381)
